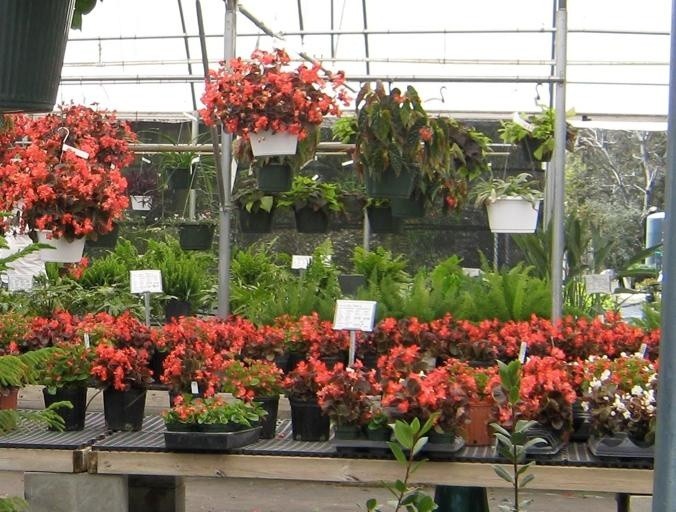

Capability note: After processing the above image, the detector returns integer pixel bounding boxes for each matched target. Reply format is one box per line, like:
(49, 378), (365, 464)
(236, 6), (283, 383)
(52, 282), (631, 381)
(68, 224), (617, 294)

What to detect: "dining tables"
(125, 168), (156, 211)
(341, 181), (366, 213)
(275, 175), (347, 233)
(0, 0), (96, 113)
(137, 238), (205, 323)
(498, 105), (576, 163)
(230, 179), (276, 234)
(470, 163), (545, 235)
(137, 131), (216, 209)
(331, 116), (363, 178)
(359, 184), (404, 233)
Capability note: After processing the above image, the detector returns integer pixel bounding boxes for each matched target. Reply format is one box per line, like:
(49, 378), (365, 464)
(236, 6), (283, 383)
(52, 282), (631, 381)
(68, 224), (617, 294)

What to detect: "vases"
(491, 314), (561, 360)
(221, 358), (284, 399)
(159, 393), (208, 424)
(282, 352), (335, 401)
(424, 312), (463, 358)
(572, 351), (658, 443)
(244, 325), (285, 361)
(91, 310), (162, 392)
(233, 125), (319, 177)
(423, 120), (469, 215)
(1, 112), (34, 160)
(379, 313), (429, 354)
(641, 328), (662, 372)
(205, 317), (256, 360)
(465, 365), (501, 404)
(322, 321), (367, 351)
(352, 80), (431, 182)
(283, 310), (322, 361)
(198, 48), (352, 141)
(23, 307), (94, 395)
(408, 356), (478, 433)
(64, 256), (89, 282)
(0, 140), (130, 244)
(159, 317), (221, 397)
(197, 396), (259, 427)
(483, 347), (574, 420)
(557, 316), (615, 357)
(309, 357), (382, 424)
(0, 312), (33, 354)
(378, 345), (424, 414)
(601, 309), (644, 357)
(363, 408), (388, 430)
(26, 102), (135, 171)
(449, 317), (503, 368)
(154, 214), (217, 232)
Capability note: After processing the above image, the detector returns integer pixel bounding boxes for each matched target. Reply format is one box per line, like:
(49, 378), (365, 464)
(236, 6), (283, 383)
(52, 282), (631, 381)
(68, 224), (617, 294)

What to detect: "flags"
(201, 425), (240, 433)
(462, 401), (494, 446)
(251, 162), (293, 192)
(237, 396), (279, 438)
(33, 229), (86, 263)
(0, 384), (20, 431)
(332, 424), (358, 440)
(165, 422), (201, 431)
(526, 411), (566, 446)
(604, 415), (653, 441)
(169, 391), (203, 409)
(360, 423), (390, 441)
(85, 221), (119, 250)
(361, 161), (418, 200)
(398, 415), (425, 444)
(289, 400), (330, 442)
(102, 388), (146, 431)
(177, 224), (215, 252)
(572, 398), (596, 439)
(430, 423), (457, 445)
(391, 188), (427, 220)
(244, 129), (296, 156)
(42, 386), (87, 431)
